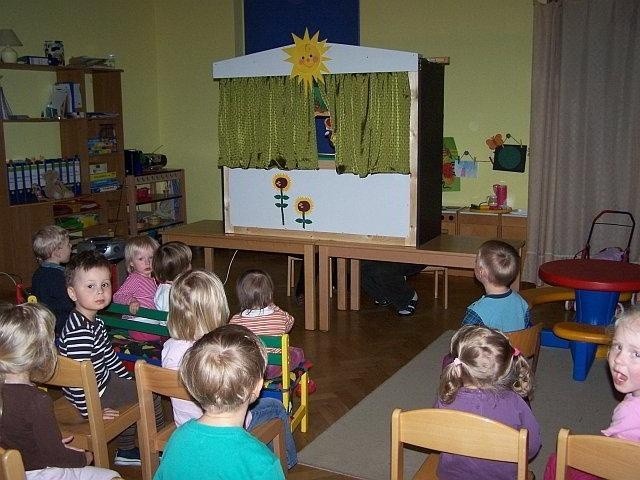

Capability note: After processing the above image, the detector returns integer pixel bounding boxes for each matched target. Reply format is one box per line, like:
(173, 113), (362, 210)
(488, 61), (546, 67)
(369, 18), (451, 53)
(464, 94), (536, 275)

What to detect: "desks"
(159, 219), (320, 331)
(310, 234), (526, 335)
(536, 257), (639, 383)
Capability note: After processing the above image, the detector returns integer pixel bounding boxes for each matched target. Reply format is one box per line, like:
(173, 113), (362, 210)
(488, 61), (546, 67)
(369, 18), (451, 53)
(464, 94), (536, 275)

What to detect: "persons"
(31, 223), (75, 333)
(543, 303), (640, 480)
(112, 235), (161, 343)
(150, 325), (285, 479)
(153, 241), (194, 327)
(59, 250), (166, 465)
(161, 267), (299, 470)
(461, 240), (532, 334)
(437, 323), (542, 480)
(361, 261), (427, 316)
(229, 271), (318, 398)
(293, 254), (338, 304)
(0, 301), (123, 480)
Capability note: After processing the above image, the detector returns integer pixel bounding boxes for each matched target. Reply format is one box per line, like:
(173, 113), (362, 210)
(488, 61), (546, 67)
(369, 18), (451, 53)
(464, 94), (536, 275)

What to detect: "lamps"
(0, 28), (24, 63)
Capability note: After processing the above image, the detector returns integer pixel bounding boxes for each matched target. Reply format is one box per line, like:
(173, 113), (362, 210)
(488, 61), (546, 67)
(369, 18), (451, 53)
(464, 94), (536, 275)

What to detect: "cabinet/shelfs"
(119, 165), (191, 241)
(441, 212), (528, 282)
(0, 61), (123, 291)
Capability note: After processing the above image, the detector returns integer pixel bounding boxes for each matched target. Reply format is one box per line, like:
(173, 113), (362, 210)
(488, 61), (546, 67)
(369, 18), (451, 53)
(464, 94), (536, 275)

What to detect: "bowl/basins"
(137, 187), (149, 200)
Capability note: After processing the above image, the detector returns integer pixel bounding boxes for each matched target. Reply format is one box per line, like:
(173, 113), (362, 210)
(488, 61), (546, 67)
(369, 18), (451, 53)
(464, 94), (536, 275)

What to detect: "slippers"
(396, 300), (417, 316)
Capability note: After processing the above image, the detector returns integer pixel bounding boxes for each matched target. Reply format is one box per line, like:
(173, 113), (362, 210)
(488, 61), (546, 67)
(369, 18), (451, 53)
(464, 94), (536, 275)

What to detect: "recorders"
(143, 153), (168, 168)
(77, 237), (125, 264)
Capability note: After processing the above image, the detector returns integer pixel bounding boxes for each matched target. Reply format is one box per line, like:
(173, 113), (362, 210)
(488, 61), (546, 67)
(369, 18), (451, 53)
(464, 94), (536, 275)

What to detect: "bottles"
(108, 54), (115, 69)
(487, 182), (509, 209)
(135, 212), (162, 231)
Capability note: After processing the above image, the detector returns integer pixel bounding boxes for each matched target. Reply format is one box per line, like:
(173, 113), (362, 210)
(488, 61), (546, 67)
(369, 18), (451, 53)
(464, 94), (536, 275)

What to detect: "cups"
(162, 189), (169, 198)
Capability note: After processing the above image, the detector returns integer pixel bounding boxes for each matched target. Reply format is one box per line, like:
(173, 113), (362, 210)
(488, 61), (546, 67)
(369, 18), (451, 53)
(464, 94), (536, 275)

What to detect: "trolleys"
(563, 209), (637, 322)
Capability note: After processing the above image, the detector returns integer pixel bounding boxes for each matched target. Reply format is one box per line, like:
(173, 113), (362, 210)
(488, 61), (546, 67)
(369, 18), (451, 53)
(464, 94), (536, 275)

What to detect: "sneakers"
(296, 380), (316, 396)
(114, 447), (163, 465)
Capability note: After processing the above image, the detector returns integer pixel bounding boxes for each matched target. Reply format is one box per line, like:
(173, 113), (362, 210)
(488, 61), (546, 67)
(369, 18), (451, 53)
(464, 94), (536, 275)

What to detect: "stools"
(284, 252), (450, 315)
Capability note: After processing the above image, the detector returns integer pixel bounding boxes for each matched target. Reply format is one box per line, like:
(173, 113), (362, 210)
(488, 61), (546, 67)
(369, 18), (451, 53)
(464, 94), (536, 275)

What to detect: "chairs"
(386, 407), (536, 479)
(554, 425), (640, 479)
(1, 296), (312, 479)
(504, 321), (544, 379)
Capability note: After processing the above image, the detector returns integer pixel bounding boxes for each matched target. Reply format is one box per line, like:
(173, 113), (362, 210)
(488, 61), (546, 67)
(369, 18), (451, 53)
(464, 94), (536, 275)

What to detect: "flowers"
(293, 196), (315, 230)
(271, 172), (292, 225)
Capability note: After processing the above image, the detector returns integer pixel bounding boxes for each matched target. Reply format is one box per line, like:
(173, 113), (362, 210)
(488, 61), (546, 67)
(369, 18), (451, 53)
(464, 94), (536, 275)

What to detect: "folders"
(7, 158), (81, 205)
